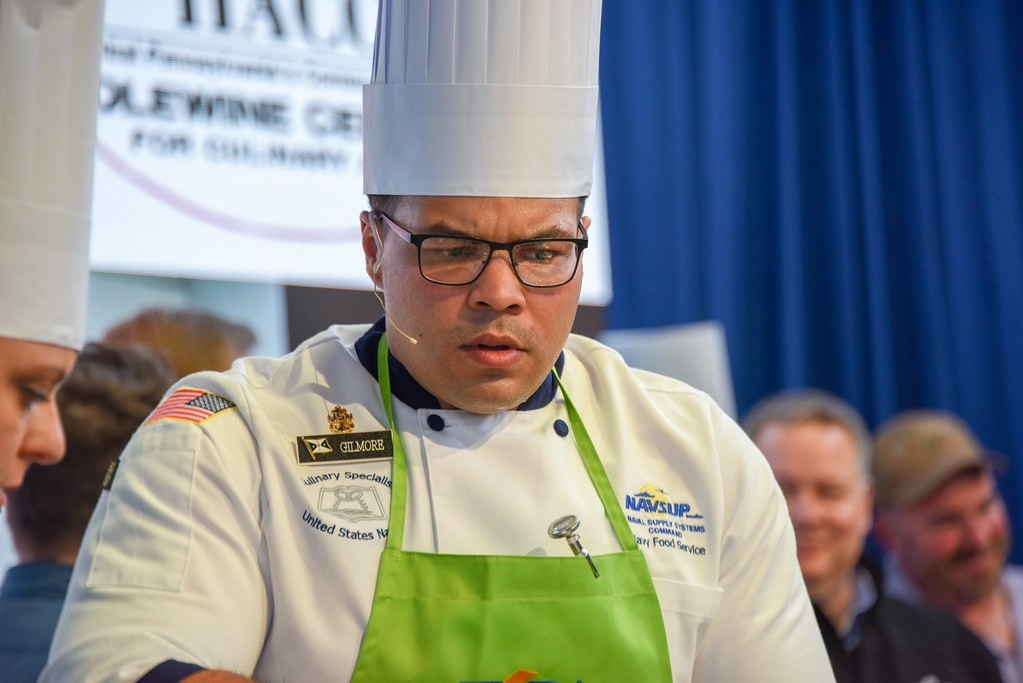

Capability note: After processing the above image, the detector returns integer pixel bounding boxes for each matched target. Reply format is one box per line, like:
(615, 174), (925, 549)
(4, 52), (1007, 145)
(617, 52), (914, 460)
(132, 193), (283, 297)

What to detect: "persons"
(38, 193), (838, 683)
(0, 342), (181, 683)
(870, 416), (1022, 683)
(736, 389), (1004, 683)
(0, 334), (104, 516)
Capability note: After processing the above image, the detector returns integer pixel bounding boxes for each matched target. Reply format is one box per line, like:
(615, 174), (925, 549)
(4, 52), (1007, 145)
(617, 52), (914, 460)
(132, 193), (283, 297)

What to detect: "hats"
(868, 414), (986, 509)
(362, 0), (602, 198)
(0, 0), (103, 354)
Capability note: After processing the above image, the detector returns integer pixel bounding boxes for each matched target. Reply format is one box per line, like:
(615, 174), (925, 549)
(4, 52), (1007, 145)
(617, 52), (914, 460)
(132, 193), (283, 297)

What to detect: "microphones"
(373, 275), (417, 345)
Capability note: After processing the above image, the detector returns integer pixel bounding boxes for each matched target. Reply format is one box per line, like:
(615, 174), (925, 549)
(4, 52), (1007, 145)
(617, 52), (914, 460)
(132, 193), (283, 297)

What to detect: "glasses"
(373, 211), (588, 288)
